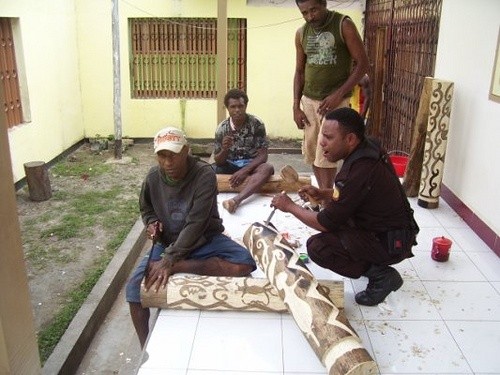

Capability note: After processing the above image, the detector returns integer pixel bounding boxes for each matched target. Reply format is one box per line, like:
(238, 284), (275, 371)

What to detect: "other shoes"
(223, 198), (237, 216)
(356, 267), (402, 307)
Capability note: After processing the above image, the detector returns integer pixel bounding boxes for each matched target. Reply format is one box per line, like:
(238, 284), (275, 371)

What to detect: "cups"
(431, 236), (453, 261)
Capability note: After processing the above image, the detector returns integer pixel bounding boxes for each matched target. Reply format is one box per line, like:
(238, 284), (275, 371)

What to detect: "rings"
(326, 107), (329, 110)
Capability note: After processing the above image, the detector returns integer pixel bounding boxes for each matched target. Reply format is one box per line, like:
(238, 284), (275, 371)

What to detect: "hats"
(154, 124), (186, 155)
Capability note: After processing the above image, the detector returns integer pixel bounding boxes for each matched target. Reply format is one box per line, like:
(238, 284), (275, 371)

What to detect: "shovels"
(281, 165), (318, 207)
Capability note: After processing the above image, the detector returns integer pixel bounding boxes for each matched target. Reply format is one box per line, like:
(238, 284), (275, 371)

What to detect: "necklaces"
(312, 12), (331, 37)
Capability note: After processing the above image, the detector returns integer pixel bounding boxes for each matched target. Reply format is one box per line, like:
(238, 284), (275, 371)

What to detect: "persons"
(125, 127), (257, 350)
(208, 89), (275, 214)
(270, 107), (420, 306)
(292, 0), (368, 208)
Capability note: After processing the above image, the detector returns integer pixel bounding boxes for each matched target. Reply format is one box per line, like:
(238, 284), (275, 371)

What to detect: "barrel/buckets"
(387, 150), (409, 178)
